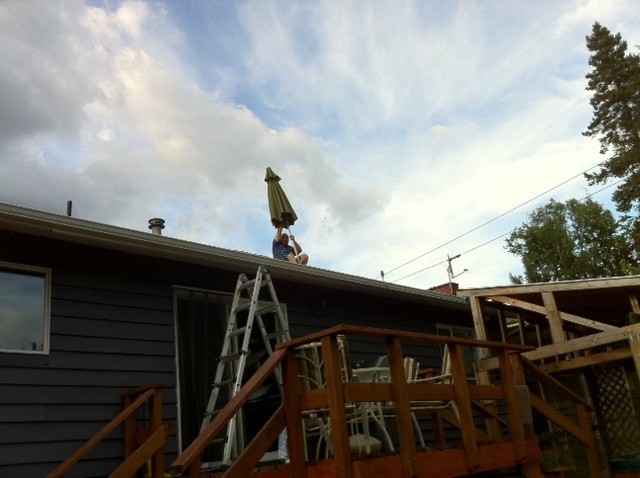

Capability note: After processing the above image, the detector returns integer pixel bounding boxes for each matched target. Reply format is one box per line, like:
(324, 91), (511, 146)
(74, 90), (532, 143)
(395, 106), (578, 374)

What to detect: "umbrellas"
(264, 166), (302, 264)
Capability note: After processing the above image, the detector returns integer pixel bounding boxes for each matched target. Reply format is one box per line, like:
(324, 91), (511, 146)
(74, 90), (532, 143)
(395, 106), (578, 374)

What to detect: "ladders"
(200, 265), (334, 471)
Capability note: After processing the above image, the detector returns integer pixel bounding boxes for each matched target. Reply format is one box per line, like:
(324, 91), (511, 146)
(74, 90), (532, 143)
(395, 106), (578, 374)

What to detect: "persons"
(272, 223), (308, 265)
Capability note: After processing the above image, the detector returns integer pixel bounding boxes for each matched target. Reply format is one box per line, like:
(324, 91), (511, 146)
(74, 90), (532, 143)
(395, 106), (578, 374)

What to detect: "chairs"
(294, 335), (461, 462)
